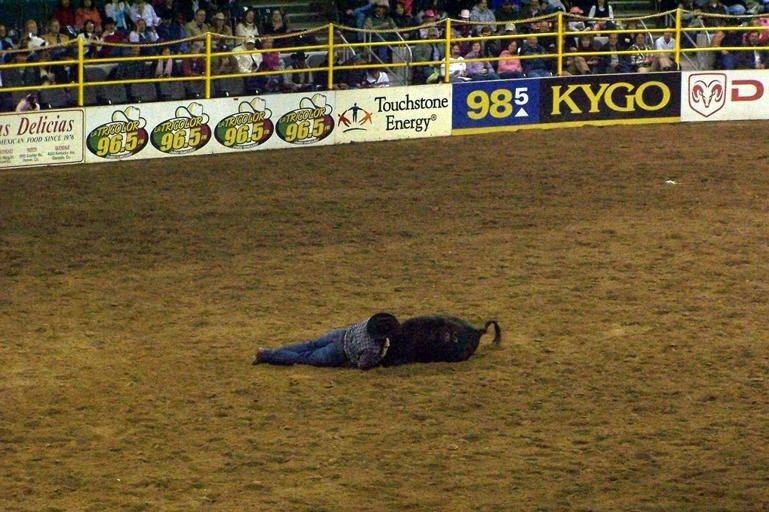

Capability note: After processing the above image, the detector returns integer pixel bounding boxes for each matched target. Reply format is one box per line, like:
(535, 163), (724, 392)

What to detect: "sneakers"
(251, 347), (268, 368)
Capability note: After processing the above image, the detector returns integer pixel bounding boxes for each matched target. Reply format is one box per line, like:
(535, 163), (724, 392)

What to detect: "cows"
(380, 314), (501, 368)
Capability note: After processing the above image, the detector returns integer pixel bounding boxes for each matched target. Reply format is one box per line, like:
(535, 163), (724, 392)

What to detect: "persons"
(249, 312), (400, 371)
(2, 0), (769, 114)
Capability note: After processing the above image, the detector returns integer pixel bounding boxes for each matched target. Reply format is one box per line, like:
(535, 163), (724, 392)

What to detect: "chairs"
(0, 0), (769, 110)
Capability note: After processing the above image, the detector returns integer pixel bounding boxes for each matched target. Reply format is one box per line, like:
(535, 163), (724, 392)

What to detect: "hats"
(244, 33), (256, 43)
(427, 27), (440, 36)
(457, 8), (473, 18)
(100, 16), (118, 26)
(289, 50), (310, 59)
(420, 9), (435, 18)
(504, 22), (516, 31)
(210, 11), (228, 21)
(569, 5), (584, 13)
(364, 312), (404, 340)
(726, 3), (746, 13)
